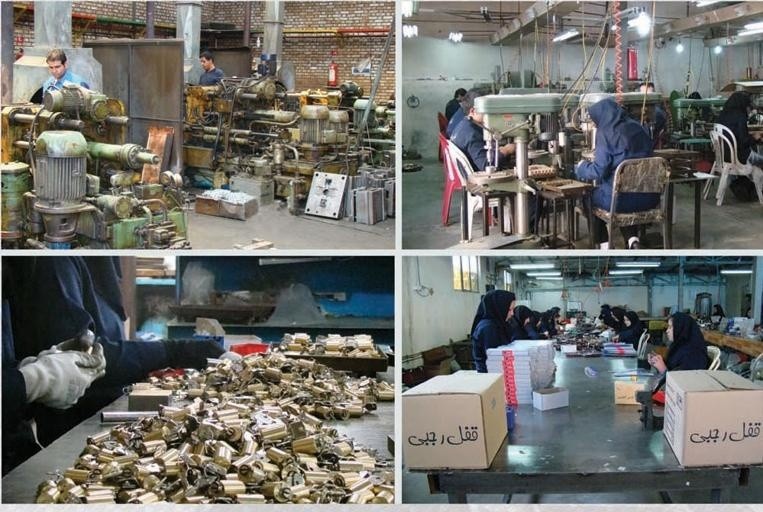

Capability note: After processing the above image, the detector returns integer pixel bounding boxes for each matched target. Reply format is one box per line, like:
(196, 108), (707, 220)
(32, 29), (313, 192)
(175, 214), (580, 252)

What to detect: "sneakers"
(599, 242), (609, 249)
(627, 236), (640, 249)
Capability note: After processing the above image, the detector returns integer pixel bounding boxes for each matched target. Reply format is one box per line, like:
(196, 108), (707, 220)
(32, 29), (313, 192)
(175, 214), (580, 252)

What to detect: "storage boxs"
(192, 183), (257, 220)
(343, 169), (394, 226)
(401, 372), (508, 472)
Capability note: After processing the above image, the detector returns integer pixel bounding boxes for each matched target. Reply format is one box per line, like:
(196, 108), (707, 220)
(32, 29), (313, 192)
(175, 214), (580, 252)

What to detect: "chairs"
(432, 106), (762, 250)
(426, 312), (762, 504)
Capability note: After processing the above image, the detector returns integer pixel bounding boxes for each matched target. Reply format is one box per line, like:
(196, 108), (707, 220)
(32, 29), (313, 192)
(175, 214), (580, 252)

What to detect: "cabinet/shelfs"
(118, 257), (180, 343)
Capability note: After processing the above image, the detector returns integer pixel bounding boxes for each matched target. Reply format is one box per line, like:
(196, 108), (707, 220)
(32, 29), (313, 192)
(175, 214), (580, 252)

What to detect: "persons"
(3, 254), (108, 477)
(575, 97), (661, 249)
(745, 307), (751, 317)
(20, 255), (244, 448)
(508, 305), (562, 341)
(647, 310), (707, 385)
(40, 47), (89, 94)
(598, 303), (644, 352)
(445, 92), (484, 143)
(470, 289), (516, 373)
(445, 87), (467, 120)
(447, 90), (542, 234)
(198, 50), (228, 85)
(711, 303), (726, 318)
(718, 91), (763, 202)
(640, 83), (670, 142)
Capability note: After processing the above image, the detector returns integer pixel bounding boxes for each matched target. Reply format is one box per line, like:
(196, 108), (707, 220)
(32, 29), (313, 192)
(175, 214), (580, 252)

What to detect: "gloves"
(18, 330), (105, 411)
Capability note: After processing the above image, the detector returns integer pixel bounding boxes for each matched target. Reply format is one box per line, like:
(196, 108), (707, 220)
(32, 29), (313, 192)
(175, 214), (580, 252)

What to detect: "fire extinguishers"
(628, 48), (637, 80)
(328, 61), (338, 86)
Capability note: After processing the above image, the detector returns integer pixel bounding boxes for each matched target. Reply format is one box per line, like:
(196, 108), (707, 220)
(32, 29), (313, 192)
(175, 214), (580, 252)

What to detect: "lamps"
(508, 256), (753, 281)
(402, 1), (763, 55)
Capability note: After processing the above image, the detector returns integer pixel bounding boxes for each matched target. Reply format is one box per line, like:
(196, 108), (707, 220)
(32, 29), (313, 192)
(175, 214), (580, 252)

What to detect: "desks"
(165, 315), (394, 350)
(1, 384), (393, 502)
